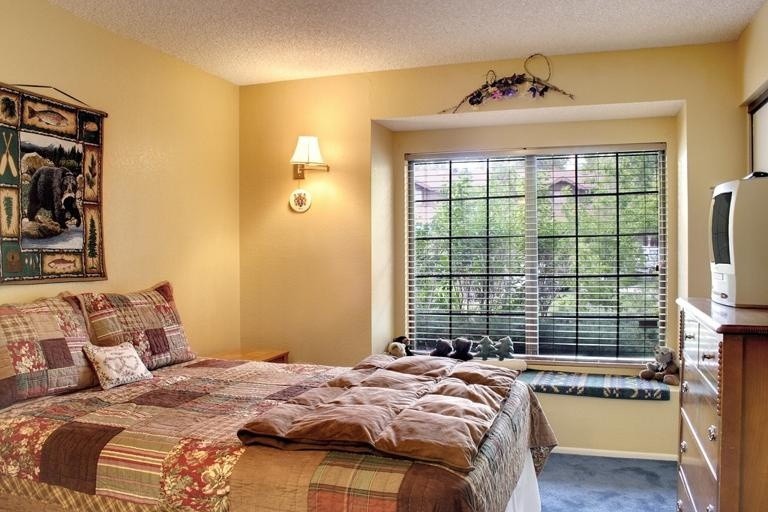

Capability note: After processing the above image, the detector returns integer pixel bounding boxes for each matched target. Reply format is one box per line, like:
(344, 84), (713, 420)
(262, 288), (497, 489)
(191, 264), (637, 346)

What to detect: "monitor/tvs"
(708, 178), (768, 310)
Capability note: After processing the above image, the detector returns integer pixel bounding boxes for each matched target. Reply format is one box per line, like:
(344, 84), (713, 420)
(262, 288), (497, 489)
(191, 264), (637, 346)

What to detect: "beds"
(0, 357), (558, 512)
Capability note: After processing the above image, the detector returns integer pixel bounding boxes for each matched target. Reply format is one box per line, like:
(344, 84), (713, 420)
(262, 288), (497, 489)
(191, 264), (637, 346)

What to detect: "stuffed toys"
(639, 345), (679, 386)
(430, 338), (453, 356)
(388, 341), (407, 357)
(449, 338), (473, 361)
(393, 335), (415, 356)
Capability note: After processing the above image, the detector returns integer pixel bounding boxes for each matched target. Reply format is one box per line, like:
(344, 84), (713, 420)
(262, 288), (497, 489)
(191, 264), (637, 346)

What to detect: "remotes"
(742, 172), (768, 179)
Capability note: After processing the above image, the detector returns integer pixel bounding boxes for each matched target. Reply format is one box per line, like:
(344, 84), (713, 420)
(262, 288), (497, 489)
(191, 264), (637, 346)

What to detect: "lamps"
(289, 136), (329, 179)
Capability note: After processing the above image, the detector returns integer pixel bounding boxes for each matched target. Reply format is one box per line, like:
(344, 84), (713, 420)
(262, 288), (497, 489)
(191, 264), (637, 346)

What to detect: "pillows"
(0, 289), (101, 408)
(62, 280), (198, 371)
(82, 341), (154, 391)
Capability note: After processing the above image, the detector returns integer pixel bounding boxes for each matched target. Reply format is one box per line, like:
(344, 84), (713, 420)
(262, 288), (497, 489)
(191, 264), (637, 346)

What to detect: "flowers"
(469, 73), (550, 110)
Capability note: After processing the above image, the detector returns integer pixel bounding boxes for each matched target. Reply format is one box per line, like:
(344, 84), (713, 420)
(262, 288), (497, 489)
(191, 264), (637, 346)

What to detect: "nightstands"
(206, 347), (293, 365)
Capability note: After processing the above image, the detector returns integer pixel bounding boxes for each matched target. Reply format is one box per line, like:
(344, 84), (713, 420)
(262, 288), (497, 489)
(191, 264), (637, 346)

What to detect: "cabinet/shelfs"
(675, 297), (768, 512)
